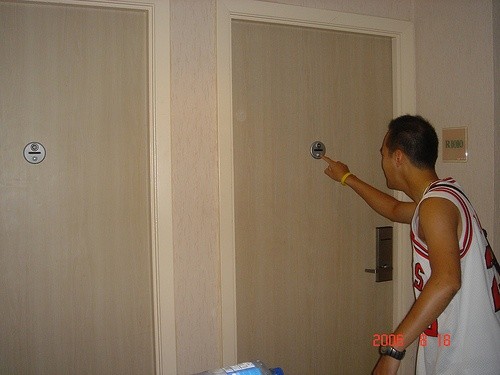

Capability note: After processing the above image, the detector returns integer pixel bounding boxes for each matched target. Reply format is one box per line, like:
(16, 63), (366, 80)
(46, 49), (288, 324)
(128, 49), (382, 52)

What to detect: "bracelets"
(341, 172), (352, 185)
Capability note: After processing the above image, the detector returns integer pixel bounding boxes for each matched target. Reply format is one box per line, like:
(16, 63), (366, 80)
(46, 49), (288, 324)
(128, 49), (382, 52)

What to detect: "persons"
(319, 113), (499, 375)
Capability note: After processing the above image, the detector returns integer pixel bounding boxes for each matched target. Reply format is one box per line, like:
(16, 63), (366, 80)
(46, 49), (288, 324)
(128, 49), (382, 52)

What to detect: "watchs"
(378, 342), (407, 361)
